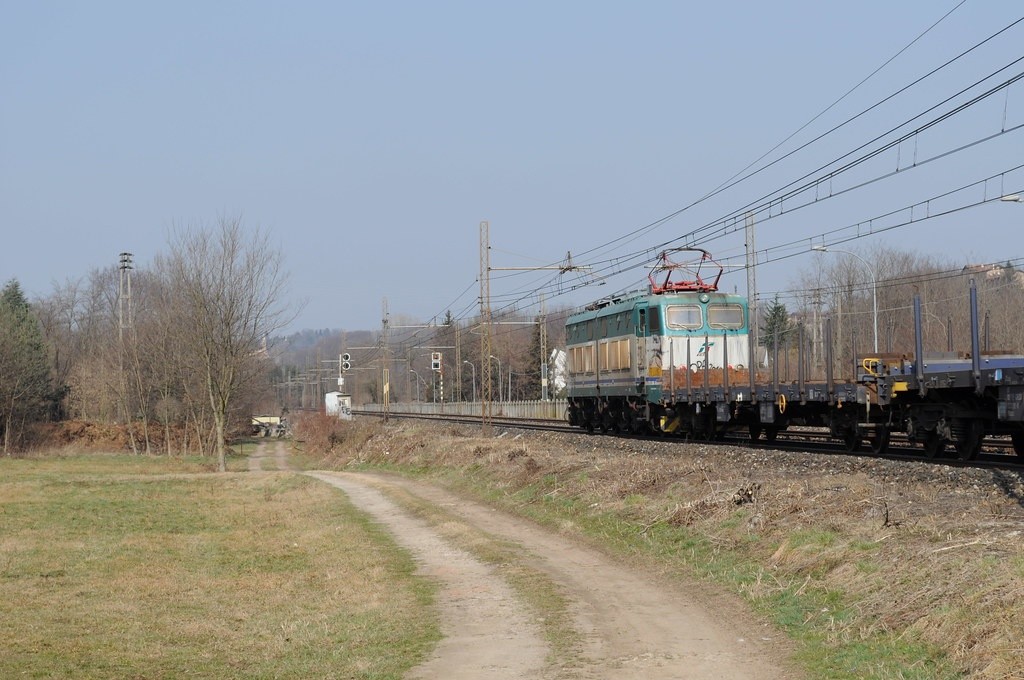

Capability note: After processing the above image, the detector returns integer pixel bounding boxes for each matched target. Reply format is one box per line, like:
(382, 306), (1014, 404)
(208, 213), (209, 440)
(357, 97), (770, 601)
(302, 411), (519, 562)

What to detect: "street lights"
(464, 360), (476, 402)
(425, 367), (435, 403)
(813, 246), (879, 353)
(409, 369), (419, 402)
(490, 355), (502, 402)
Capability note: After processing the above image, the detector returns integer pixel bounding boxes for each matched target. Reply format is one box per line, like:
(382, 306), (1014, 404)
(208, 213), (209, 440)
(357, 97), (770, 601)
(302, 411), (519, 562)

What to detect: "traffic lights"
(342, 353), (350, 370)
(432, 353), (440, 369)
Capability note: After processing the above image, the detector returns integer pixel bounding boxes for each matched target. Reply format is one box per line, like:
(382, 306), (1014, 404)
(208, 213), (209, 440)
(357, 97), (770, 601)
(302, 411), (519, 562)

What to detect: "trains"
(563, 288), (1024, 459)
